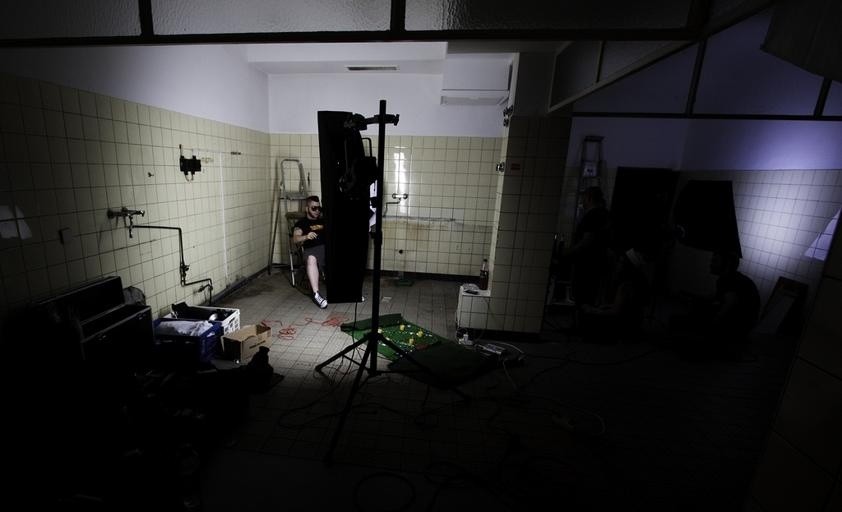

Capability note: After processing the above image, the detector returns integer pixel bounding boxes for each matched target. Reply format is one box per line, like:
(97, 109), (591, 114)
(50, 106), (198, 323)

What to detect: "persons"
(290, 196), (367, 309)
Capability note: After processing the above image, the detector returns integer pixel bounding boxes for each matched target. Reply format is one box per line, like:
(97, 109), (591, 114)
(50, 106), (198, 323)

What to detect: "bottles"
(479, 259), (489, 290)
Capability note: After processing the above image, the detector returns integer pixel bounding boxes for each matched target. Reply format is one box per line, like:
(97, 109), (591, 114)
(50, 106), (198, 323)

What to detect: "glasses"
(306, 205), (322, 212)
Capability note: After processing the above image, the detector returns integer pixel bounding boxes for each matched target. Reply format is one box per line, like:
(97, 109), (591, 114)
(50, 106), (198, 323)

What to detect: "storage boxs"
(156, 303), (239, 368)
(219, 321), (273, 362)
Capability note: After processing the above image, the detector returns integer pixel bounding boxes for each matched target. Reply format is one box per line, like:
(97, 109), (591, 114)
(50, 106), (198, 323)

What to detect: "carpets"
(340, 312), (484, 386)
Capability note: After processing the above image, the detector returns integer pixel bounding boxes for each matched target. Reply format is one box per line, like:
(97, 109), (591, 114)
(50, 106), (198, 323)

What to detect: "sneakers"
(313, 292), (329, 309)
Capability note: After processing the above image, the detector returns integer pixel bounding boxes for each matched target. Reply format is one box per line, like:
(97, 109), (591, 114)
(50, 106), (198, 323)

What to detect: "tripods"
(315, 100), (473, 466)
